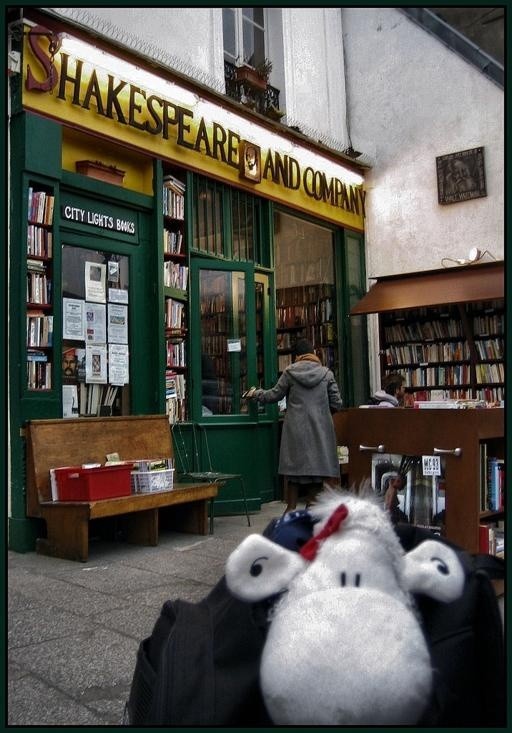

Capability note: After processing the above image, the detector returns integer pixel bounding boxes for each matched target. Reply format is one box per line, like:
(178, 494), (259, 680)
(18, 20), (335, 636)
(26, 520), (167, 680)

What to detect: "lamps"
(439, 245), (496, 269)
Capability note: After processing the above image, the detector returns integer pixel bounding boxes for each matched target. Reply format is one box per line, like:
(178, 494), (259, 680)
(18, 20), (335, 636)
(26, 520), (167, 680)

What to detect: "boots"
(284, 487), (316, 513)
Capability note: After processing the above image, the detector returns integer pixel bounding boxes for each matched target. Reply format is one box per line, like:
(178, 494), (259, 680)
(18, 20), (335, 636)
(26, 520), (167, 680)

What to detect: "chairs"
(165, 418), (252, 533)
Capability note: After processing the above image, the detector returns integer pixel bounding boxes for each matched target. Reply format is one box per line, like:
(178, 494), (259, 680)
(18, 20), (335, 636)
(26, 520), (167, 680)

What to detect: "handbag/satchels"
(124, 510), (507, 728)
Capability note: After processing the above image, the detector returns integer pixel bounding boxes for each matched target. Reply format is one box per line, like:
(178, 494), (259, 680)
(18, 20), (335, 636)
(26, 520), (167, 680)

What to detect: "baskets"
(130, 468), (176, 495)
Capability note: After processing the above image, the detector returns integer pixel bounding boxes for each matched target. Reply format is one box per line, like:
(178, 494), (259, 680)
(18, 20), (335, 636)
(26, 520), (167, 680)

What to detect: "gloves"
(241, 387), (257, 399)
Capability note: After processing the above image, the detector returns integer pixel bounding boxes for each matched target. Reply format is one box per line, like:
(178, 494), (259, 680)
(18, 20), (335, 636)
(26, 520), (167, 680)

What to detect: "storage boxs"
(50, 463), (134, 503)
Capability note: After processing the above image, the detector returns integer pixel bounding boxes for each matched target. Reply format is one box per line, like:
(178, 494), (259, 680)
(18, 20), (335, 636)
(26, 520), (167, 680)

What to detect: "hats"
(295, 339), (314, 356)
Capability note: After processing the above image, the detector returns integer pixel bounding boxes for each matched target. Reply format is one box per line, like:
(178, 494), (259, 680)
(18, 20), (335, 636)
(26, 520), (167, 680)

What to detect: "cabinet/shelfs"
(192, 282), (337, 415)
(158, 168), (192, 416)
(346, 407), (504, 596)
(378, 297), (506, 407)
(22, 182), (55, 395)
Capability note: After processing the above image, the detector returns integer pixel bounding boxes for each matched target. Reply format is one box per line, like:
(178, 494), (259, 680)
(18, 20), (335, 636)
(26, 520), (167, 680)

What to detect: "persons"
(63, 353), (78, 376)
(241, 339), (343, 515)
(372, 372), (406, 405)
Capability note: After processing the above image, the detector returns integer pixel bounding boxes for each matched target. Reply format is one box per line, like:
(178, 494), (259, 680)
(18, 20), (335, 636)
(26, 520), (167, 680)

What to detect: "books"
(478, 442), (504, 559)
(26, 187), (55, 389)
(199, 269), (334, 414)
(62, 381), (124, 418)
(163, 173), (189, 423)
(381, 298), (505, 408)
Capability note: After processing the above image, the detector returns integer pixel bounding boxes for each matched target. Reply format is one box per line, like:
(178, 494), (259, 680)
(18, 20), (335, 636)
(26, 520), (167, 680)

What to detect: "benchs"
(20, 416), (220, 564)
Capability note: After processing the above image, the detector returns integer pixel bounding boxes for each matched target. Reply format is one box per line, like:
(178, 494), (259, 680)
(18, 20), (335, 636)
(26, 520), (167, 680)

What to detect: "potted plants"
(234, 50), (274, 93)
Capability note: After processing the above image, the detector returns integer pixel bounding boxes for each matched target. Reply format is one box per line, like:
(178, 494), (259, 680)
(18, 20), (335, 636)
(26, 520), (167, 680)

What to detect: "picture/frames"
(236, 135), (263, 185)
(434, 146), (489, 206)
